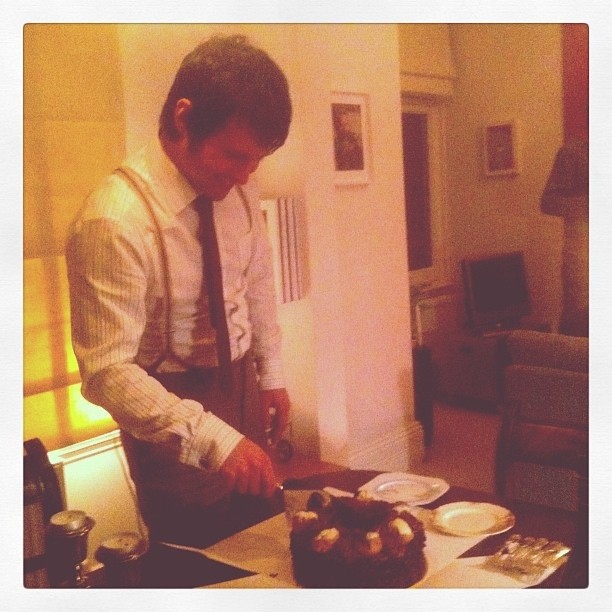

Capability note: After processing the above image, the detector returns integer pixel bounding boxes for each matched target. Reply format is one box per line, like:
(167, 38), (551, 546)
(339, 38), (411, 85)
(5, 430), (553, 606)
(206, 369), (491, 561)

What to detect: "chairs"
(492, 331), (588, 512)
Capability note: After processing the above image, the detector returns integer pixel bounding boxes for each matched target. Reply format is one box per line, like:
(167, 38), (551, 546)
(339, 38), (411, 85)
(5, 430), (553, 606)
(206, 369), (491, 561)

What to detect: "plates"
(430, 501), (517, 538)
(356, 471), (450, 507)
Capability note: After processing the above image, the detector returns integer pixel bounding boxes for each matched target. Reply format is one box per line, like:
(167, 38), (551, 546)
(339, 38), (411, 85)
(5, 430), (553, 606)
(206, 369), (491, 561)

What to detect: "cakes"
(289, 488), (430, 588)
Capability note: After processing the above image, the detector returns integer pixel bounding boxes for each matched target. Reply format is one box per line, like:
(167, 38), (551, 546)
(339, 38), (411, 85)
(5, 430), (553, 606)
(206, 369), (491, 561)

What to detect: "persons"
(541, 133), (589, 334)
(66, 37), (292, 550)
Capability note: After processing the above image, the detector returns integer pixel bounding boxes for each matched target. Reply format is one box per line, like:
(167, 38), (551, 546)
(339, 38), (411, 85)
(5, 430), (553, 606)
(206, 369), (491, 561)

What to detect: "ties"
(195, 198), (232, 399)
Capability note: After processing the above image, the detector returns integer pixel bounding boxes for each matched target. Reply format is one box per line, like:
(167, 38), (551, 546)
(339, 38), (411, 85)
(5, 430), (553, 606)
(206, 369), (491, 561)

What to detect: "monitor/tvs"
(460, 249), (533, 337)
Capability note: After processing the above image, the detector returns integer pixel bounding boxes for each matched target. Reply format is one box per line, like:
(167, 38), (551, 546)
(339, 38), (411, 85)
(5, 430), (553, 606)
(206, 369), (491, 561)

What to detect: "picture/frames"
(327, 94), (370, 187)
(483, 118), (520, 177)
